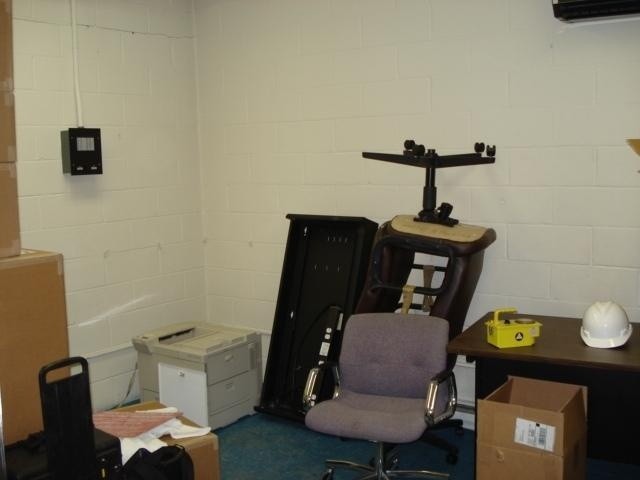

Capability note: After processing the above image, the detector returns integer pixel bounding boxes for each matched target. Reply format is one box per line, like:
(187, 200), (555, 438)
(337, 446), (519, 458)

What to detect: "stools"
(327, 284), (468, 469)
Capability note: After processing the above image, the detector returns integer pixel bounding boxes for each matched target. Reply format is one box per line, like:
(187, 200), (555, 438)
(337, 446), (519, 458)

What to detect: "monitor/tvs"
(550, 0), (640, 24)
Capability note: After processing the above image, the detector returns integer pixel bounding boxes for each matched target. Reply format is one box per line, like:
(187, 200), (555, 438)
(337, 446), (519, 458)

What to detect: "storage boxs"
(474, 371), (593, 480)
(0, 246), (73, 449)
(92, 399), (222, 480)
(1, 163), (23, 259)
(0, 0), (16, 91)
(0, 91), (19, 164)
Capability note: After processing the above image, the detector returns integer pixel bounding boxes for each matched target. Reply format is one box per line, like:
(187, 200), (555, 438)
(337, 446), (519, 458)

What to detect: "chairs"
(294, 309), (459, 479)
(355, 138), (498, 374)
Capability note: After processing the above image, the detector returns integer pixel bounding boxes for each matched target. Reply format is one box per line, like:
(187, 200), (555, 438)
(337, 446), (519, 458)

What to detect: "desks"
(445, 308), (640, 461)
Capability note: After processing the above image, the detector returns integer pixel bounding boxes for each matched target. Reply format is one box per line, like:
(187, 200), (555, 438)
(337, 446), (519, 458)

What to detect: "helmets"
(581, 301), (632, 348)
